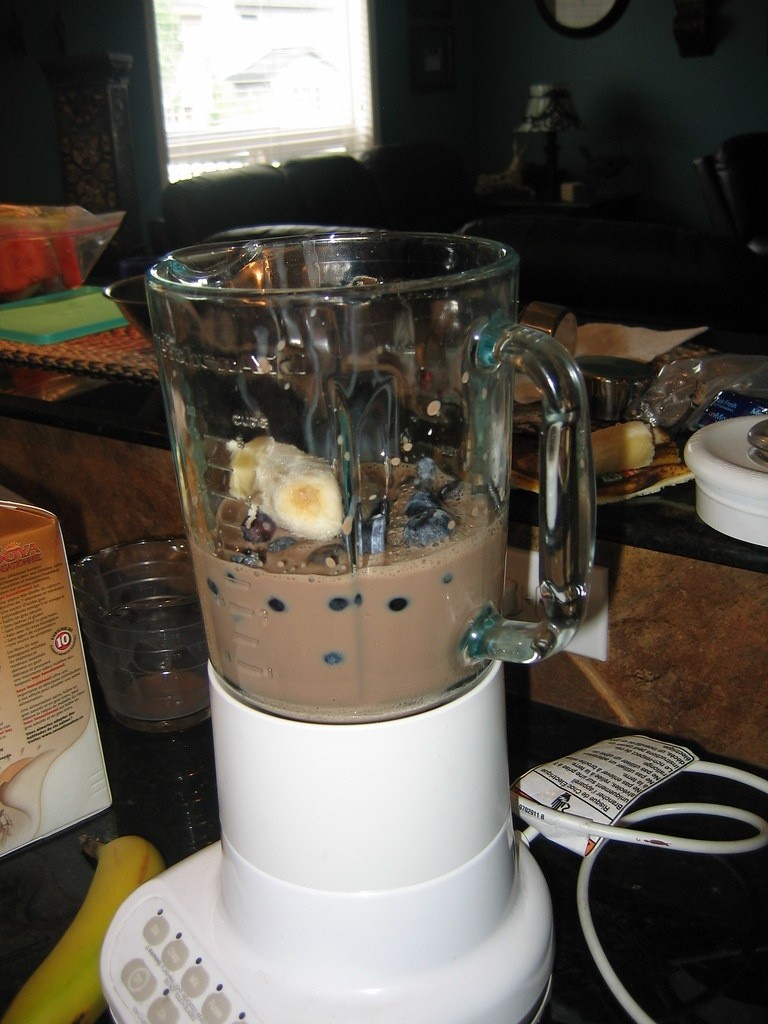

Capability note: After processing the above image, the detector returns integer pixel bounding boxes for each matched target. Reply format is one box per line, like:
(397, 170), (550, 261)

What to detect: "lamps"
(511, 81), (587, 204)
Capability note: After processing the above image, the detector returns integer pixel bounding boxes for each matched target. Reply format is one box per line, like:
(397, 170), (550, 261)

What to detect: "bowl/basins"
(103, 275), (160, 352)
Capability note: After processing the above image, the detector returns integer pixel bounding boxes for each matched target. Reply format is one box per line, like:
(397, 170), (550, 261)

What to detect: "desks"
(0, 545), (767, 1024)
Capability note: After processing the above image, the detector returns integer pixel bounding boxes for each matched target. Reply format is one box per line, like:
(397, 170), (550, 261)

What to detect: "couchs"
(145, 137), (486, 257)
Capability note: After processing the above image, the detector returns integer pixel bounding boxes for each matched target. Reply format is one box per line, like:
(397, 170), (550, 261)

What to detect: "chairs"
(446, 209), (768, 355)
(692, 129), (768, 333)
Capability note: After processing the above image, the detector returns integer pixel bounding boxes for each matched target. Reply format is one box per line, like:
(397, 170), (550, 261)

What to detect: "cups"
(70, 535), (212, 734)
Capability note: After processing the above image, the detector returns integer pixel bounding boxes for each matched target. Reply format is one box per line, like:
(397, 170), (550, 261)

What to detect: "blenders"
(101, 230), (596, 1024)
